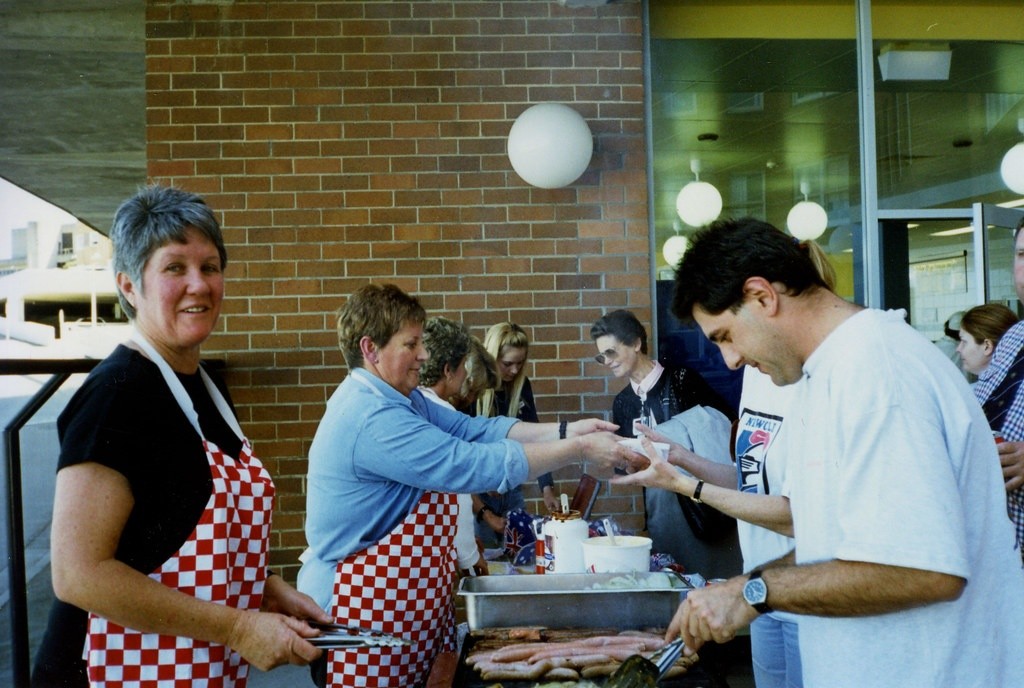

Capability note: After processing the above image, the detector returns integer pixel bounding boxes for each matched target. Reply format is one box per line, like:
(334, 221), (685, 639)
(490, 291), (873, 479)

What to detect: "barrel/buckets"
(582, 535), (653, 573)
(544, 509), (589, 574)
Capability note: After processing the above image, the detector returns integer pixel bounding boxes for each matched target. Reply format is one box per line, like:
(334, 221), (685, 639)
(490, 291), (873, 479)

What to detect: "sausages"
(466, 623), (699, 688)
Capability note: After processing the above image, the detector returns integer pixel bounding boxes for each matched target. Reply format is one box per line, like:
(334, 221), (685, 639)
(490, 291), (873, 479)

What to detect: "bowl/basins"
(621, 440), (671, 475)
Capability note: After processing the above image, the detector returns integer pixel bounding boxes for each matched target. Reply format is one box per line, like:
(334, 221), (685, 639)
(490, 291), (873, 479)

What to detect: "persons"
(607, 211), (1024, 684)
(591, 304), (744, 580)
(295, 279), (649, 687)
(31, 177), (335, 688)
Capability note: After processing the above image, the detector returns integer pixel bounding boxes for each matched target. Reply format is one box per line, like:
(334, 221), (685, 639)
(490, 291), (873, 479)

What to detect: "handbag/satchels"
(675, 490), (736, 542)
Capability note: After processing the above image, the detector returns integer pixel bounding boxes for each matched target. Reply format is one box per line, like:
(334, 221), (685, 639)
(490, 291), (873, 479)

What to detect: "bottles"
(991, 430), (1004, 443)
(536, 534), (546, 574)
(705, 578), (727, 586)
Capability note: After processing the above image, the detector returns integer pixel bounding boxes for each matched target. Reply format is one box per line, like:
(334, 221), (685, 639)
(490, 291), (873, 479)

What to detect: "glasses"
(595, 331), (636, 365)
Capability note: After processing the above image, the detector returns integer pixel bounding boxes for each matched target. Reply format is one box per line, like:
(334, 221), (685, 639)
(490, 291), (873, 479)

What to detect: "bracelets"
(264, 582), (288, 595)
